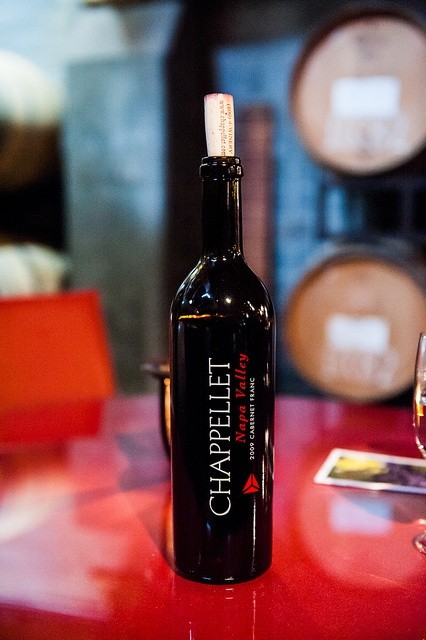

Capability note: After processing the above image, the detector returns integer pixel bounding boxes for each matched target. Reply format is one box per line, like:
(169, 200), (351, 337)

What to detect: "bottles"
(170, 95), (276, 585)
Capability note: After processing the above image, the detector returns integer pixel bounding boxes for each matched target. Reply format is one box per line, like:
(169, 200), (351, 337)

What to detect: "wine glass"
(413, 332), (426, 556)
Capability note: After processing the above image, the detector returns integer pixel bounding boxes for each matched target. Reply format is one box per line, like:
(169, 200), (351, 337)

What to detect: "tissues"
(1, 244), (113, 455)
